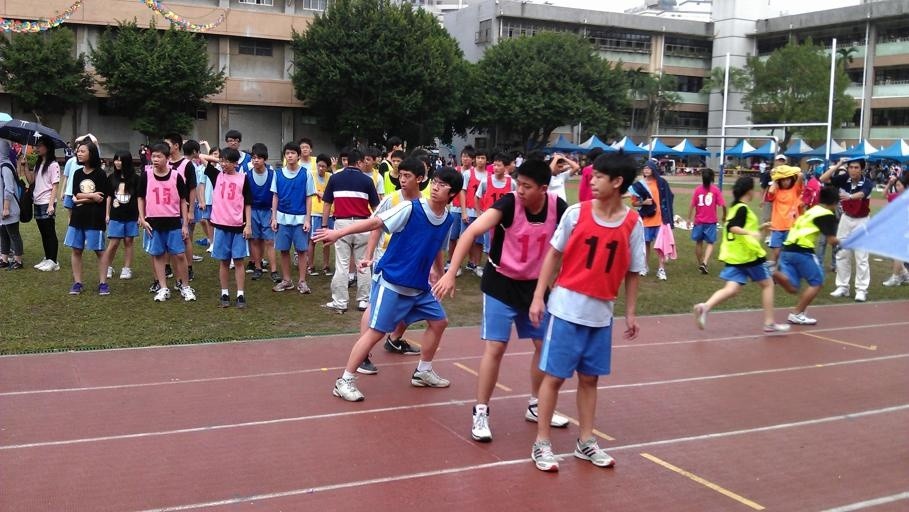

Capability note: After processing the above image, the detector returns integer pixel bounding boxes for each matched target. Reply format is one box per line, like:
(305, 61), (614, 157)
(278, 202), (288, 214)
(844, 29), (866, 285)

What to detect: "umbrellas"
(0, 119), (70, 158)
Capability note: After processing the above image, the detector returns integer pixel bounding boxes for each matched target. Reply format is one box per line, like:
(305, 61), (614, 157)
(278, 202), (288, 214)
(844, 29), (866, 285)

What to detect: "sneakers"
(830, 273), (909, 300)
(383, 335), (421, 355)
(245, 251), (333, 294)
(574, 436), (616, 467)
(98, 282), (110, 295)
(149, 255), (203, 302)
(525, 397), (570, 428)
(356, 355), (379, 375)
(787, 312), (817, 325)
(219, 294), (230, 307)
(357, 301), (369, 311)
(531, 440), (560, 472)
(445, 261), (483, 277)
(106, 266), (133, 278)
(699, 262), (708, 273)
(409, 369), (450, 388)
(333, 377), (365, 403)
(205, 243), (213, 253)
(762, 322), (791, 333)
(639, 264), (667, 280)
(693, 303), (707, 330)
(69, 282), (84, 297)
(235, 294), (245, 308)
(0, 257), (61, 271)
(322, 302), (346, 312)
(471, 405), (492, 442)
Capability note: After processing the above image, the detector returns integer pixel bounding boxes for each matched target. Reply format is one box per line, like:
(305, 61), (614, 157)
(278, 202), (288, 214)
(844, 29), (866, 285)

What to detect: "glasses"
(429, 179), (451, 191)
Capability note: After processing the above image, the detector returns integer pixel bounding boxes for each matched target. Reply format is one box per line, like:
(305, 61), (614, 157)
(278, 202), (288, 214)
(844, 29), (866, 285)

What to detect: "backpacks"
(1, 163), (35, 223)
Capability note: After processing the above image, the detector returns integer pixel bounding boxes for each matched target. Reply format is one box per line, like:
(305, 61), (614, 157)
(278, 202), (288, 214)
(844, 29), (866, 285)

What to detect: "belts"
(341, 217), (368, 220)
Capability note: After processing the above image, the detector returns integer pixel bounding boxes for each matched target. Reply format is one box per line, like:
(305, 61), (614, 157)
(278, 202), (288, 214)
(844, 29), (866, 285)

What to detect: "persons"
(529, 151), (646, 472)
(355, 159), (426, 374)
(432, 160), (570, 443)
(311, 166), (464, 403)
